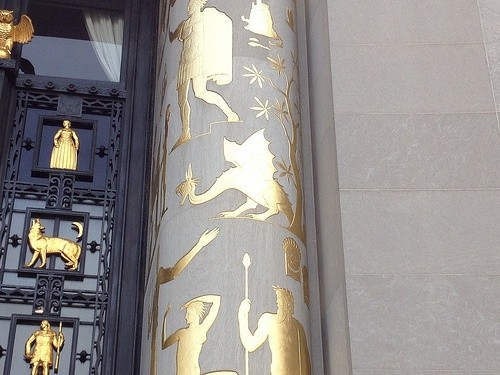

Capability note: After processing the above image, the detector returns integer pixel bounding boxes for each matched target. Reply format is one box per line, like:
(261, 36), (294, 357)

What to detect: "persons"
(50, 120), (79, 171)
(25, 319), (64, 375)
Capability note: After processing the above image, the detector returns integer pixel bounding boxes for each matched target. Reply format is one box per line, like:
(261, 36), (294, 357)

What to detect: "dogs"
(26, 217), (84, 272)
